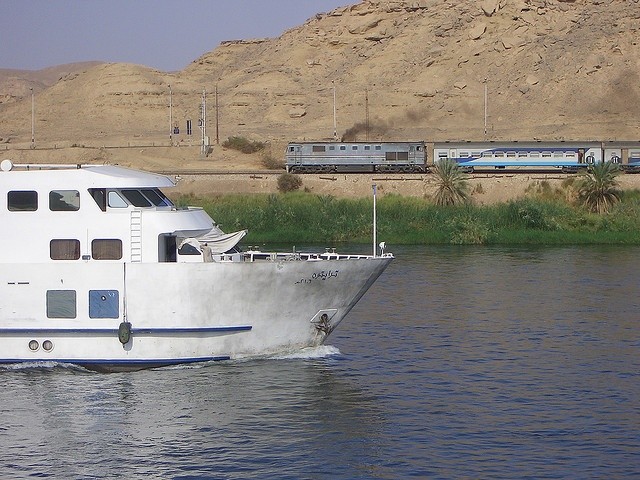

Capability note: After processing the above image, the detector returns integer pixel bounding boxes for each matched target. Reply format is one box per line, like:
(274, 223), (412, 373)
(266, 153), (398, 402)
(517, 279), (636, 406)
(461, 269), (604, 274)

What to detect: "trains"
(284, 139), (640, 173)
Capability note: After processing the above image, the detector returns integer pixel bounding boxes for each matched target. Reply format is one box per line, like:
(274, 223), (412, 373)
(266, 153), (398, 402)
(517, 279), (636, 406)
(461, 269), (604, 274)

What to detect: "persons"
(200, 243), (215, 262)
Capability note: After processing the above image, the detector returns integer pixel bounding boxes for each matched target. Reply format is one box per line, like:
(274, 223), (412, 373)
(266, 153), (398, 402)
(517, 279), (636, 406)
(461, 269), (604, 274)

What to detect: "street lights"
(484, 77), (488, 136)
(332, 80), (337, 138)
(29, 87), (35, 143)
(167, 83), (172, 140)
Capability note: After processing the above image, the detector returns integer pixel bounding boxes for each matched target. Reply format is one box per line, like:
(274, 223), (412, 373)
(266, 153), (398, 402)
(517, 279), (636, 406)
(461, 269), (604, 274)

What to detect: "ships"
(1, 161), (396, 372)
